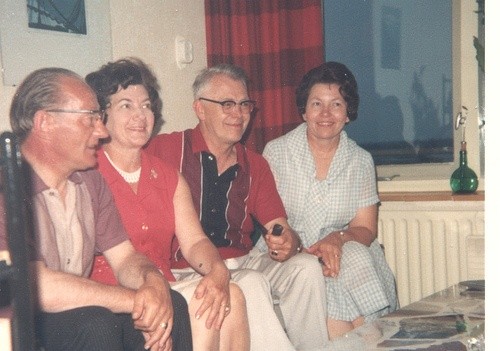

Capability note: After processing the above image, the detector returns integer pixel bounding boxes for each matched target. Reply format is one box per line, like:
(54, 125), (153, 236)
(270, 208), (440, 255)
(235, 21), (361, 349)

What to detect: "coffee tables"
(302, 280), (485, 351)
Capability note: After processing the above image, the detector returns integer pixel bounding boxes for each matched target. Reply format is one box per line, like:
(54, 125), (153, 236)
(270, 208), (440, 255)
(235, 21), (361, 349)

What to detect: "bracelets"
(335, 230), (347, 243)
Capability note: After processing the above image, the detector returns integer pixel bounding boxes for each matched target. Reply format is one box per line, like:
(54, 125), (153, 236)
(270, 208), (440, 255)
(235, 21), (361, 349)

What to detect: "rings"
(272, 250), (278, 256)
(161, 322), (168, 328)
(335, 255), (340, 257)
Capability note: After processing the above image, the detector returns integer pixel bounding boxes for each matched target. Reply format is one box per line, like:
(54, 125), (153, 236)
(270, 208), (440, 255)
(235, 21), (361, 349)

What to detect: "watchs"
(292, 229), (303, 253)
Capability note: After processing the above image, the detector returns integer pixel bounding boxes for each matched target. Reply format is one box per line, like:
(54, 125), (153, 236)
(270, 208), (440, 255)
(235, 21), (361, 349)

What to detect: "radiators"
(375, 202), (485, 308)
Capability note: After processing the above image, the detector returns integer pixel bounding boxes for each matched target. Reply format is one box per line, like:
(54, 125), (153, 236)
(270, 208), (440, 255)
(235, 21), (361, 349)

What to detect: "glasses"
(42, 109), (109, 127)
(199, 97), (256, 114)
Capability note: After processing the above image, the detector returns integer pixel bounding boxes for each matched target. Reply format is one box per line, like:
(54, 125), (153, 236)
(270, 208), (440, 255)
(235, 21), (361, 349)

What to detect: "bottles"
(450, 141), (478, 194)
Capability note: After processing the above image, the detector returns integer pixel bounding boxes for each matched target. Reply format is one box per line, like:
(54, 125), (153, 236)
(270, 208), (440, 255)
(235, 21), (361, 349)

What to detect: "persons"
(87, 57), (251, 351)
(262, 61), (402, 341)
(143, 63), (328, 351)
(0, 68), (193, 351)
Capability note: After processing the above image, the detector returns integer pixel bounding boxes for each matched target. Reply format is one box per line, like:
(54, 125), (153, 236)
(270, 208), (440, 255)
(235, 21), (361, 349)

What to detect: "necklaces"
(104, 151), (141, 183)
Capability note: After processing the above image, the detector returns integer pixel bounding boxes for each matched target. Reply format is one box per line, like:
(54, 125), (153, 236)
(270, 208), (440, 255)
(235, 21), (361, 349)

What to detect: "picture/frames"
(27, 0), (88, 36)
